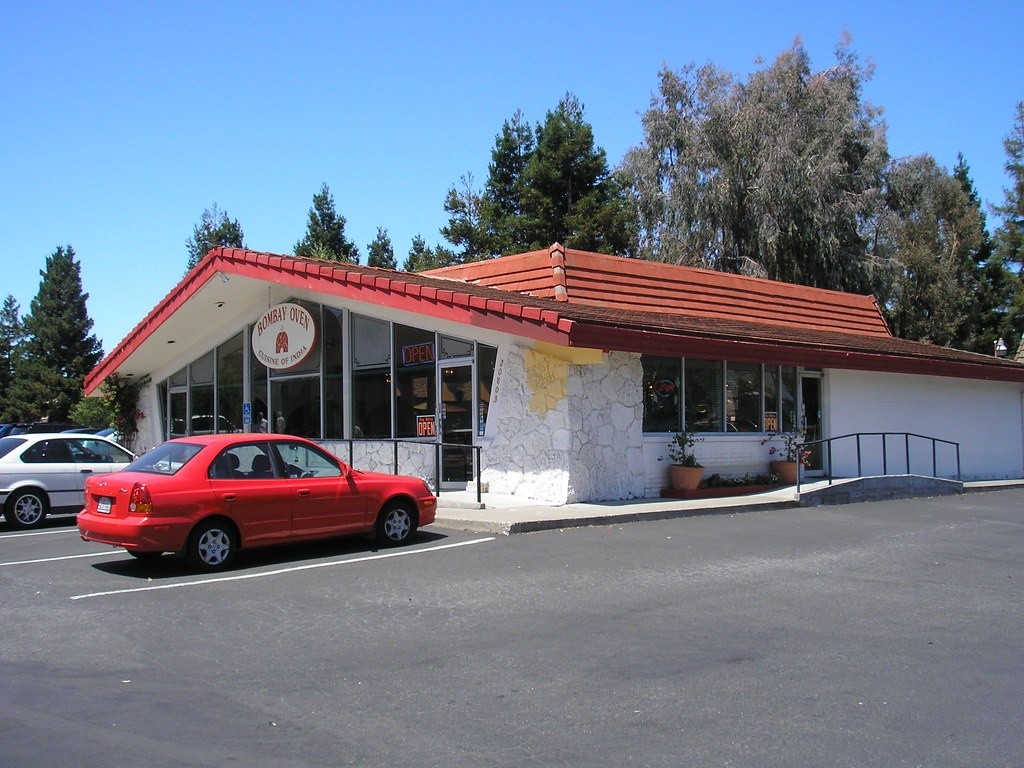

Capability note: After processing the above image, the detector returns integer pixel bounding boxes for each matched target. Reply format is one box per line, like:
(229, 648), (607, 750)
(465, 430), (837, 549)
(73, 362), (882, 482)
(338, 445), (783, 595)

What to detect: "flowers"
(759, 427), (811, 467)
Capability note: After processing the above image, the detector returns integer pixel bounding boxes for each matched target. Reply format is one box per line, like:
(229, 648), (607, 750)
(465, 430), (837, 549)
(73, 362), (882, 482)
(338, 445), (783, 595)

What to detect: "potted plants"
(658, 428), (704, 490)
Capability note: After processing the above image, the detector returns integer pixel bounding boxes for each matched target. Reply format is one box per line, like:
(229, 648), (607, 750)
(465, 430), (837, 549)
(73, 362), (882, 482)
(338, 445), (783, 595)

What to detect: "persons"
(276, 411), (285, 433)
(253, 411), (267, 432)
(353, 425), (363, 438)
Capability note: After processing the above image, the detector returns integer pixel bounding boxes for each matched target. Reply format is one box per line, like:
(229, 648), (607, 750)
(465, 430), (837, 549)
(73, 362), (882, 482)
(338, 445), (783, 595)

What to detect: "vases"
(771, 461), (797, 485)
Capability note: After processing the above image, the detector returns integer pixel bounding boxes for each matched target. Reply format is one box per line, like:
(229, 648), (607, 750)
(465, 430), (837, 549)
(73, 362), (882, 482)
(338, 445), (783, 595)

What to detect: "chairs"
(222, 454), (244, 478)
(247, 455), (273, 478)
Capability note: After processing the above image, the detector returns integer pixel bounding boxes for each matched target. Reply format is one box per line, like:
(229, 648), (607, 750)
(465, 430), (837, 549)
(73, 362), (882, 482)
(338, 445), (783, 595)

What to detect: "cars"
(160, 414), (244, 444)
(76, 432), (437, 574)
(0, 432), (184, 531)
(0, 419), (126, 464)
